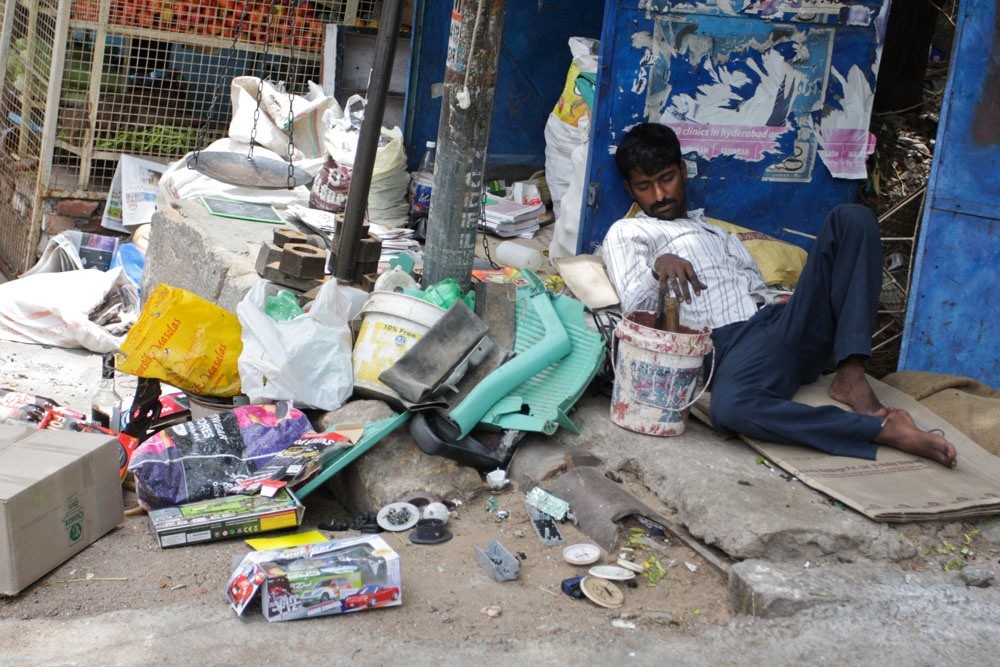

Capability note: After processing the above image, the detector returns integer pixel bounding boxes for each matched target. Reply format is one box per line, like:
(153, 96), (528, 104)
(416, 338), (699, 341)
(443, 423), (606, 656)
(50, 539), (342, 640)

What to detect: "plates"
(408, 530), (453, 544)
(376, 502), (420, 531)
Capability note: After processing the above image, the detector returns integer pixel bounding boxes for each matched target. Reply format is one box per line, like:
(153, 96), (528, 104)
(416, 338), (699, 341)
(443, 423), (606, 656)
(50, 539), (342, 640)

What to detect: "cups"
(416, 518), (444, 539)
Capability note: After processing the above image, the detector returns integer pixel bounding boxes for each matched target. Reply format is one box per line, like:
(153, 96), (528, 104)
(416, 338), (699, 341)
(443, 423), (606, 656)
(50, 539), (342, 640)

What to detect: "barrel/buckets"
(351, 289), (447, 409)
(609, 309), (715, 437)
(406, 171), (434, 218)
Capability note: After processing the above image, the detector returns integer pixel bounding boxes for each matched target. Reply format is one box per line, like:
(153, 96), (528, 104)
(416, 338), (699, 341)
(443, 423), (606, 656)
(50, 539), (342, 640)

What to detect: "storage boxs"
(148, 488), (401, 623)
(0, 422), (125, 596)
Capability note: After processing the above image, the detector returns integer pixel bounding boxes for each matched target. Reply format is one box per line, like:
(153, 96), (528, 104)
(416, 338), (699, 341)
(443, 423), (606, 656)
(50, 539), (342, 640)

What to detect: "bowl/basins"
(181, 388), (236, 421)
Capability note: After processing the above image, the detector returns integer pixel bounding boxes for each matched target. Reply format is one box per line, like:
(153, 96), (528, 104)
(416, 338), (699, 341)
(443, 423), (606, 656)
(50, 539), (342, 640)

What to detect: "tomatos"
(70, 0), (329, 52)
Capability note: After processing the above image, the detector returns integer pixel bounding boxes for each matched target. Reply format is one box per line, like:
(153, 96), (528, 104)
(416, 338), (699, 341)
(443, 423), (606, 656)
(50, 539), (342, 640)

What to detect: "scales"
(185, 1), (313, 190)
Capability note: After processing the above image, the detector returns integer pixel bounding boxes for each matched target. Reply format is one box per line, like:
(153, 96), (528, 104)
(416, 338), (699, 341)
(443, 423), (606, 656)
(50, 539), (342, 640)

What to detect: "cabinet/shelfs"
(324, 24), (410, 128)
(0, 1), (322, 192)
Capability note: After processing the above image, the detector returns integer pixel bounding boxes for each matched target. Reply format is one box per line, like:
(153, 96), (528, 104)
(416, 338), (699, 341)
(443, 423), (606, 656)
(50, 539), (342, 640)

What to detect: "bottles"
(495, 240), (542, 273)
(418, 141), (436, 178)
(92, 355), (122, 432)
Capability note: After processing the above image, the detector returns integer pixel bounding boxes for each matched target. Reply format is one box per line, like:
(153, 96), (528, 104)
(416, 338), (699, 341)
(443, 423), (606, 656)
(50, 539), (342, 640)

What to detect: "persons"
(600, 122), (959, 469)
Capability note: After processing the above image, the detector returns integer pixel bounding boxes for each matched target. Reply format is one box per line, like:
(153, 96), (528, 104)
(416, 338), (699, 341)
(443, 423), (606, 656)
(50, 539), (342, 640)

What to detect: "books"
(481, 189), (546, 236)
(287, 203), (420, 260)
